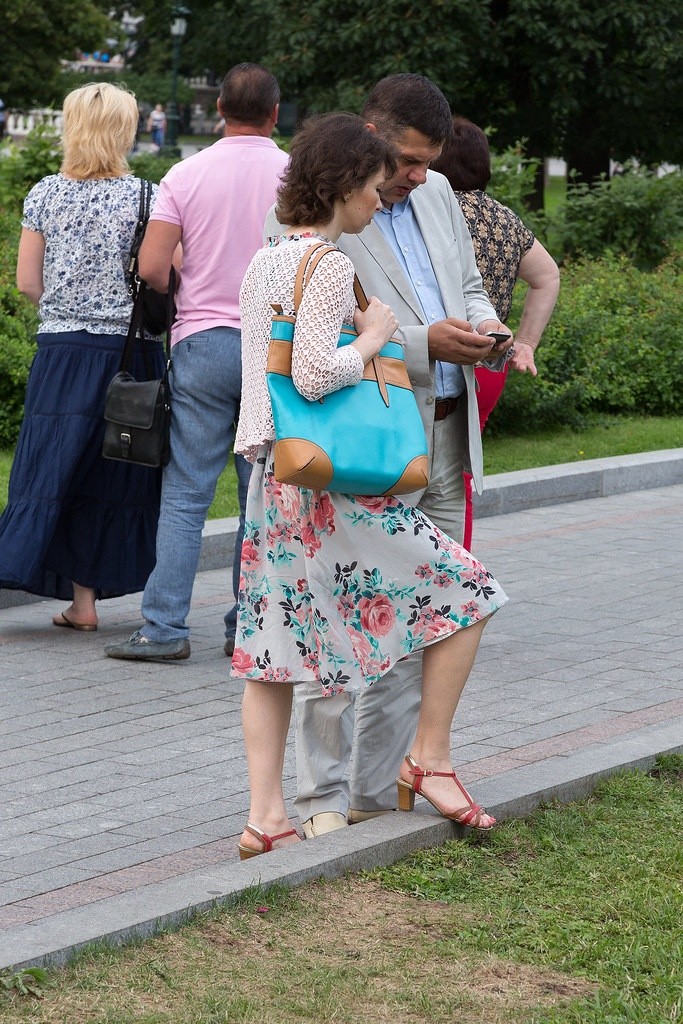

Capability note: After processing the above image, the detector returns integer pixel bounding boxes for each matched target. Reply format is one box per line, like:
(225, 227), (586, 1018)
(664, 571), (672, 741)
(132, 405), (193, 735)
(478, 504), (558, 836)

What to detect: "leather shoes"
(346, 808), (396, 826)
(298, 812), (349, 839)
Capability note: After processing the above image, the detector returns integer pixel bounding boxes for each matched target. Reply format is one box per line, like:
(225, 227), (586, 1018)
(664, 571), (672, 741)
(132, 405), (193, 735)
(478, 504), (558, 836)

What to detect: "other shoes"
(104, 631), (192, 661)
(223, 635), (235, 657)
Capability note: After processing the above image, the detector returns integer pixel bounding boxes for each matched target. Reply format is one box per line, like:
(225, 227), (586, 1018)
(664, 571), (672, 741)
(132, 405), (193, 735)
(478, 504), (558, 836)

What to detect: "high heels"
(240, 823), (296, 861)
(394, 755), (493, 831)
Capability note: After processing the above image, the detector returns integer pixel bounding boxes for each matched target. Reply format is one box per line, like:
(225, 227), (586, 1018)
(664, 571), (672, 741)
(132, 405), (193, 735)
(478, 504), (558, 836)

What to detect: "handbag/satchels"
(100, 372), (171, 468)
(265, 243), (429, 498)
(128, 218), (179, 336)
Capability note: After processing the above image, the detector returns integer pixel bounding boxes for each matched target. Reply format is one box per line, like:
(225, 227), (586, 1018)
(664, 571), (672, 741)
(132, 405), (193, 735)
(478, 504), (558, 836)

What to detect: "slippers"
(50, 612), (98, 631)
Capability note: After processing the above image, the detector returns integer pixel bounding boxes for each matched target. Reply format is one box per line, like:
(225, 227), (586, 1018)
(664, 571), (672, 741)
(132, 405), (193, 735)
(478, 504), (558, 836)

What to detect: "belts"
(434, 388), (467, 422)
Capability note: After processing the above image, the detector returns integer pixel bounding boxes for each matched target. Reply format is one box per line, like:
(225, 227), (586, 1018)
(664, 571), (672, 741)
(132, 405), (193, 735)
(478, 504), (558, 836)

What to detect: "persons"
(146, 103), (167, 148)
(427, 114), (561, 554)
(102, 62), (292, 660)
(229, 111), (509, 863)
(260, 72), (515, 844)
(0, 82), (186, 632)
(132, 106), (145, 152)
(213, 111), (226, 137)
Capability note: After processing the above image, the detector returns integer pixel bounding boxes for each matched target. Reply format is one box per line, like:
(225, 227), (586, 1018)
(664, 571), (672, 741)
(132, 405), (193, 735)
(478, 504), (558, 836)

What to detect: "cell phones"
(474, 332), (511, 349)
